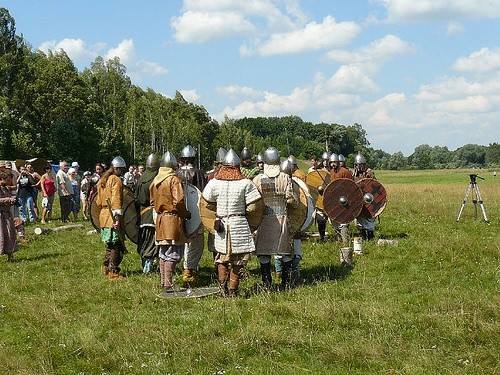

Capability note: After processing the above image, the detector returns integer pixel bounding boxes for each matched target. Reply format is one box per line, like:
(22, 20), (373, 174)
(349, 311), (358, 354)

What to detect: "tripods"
(456, 174), (488, 223)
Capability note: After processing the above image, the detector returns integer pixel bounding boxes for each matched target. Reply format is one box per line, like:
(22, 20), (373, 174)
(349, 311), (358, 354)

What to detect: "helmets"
(112, 156), (126, 168)
(322, 151), (329, 160)
(264, 147), (281, 165)
(287, 155), (297, 169)
(240, 147), (251, 160)
(330, 153), (339, 162)
(147, 153), (159, 168)
(280, 159), (291, 172)
(338, 154), (345, 163)
(257, 150), (266, 163)
(160, 151), (177, 168)
(355, 153), (366, 164)
(222, 148), (240, 166)
(181, 145), (196, 157)
(216, 147), (227, 163)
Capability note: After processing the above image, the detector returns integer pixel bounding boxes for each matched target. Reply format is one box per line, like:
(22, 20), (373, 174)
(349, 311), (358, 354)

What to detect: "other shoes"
(22, 214), (91, 226)
(18, 236), (29, 243)
(7, 254), (13, 261)
(100, 263), (301, 299)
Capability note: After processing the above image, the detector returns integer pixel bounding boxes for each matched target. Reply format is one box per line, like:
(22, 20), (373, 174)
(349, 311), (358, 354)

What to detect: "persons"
(5, 161), (42, 242)
(56, 161), (75, 223)
(149, 150), (191, 291)
(94, 155), (126, 279)
(66, 144), (379, 291)
(0, 168), (17, 263)
(41, 164), (57, 225)
(202, 147), (262, 298)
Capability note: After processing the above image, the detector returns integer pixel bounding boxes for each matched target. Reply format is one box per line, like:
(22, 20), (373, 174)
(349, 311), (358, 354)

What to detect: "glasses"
(46, 167), (51, 170)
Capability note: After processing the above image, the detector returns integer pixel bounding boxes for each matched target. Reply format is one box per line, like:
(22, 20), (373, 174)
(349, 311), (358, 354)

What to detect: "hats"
(71, 162), (79, 167)
(5, 161), (12, 168)
(83, 171), (91, 176)
